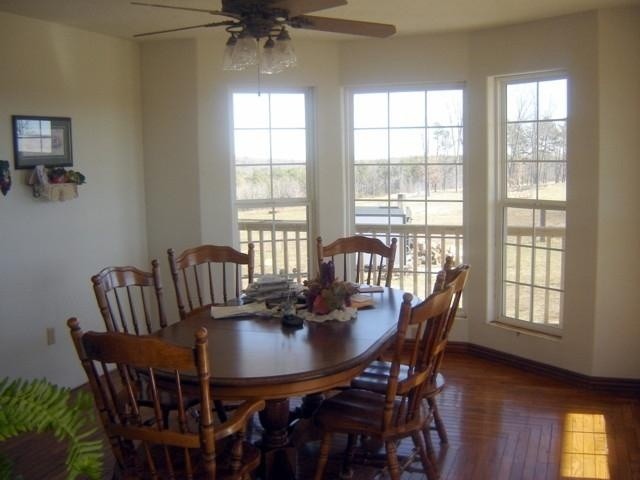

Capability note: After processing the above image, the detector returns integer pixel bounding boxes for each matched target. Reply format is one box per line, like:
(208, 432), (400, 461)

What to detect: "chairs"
(348, 257), (471, 480)
(66, 317), (266, 480)
(91, 261), (234, 468)
(167, 244), (255, 318)
(317, 234), (401, 360)
(313, 287), (455, 479)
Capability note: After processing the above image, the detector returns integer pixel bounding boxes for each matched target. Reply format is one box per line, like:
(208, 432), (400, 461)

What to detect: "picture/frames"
(12, 114), (74, 169)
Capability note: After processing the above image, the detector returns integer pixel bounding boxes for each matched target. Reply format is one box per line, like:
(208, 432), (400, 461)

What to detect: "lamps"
(221, 27), (296, 75)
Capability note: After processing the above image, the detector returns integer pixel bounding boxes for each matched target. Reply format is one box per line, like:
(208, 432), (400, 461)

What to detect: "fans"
(130, 0), (395, 39)
